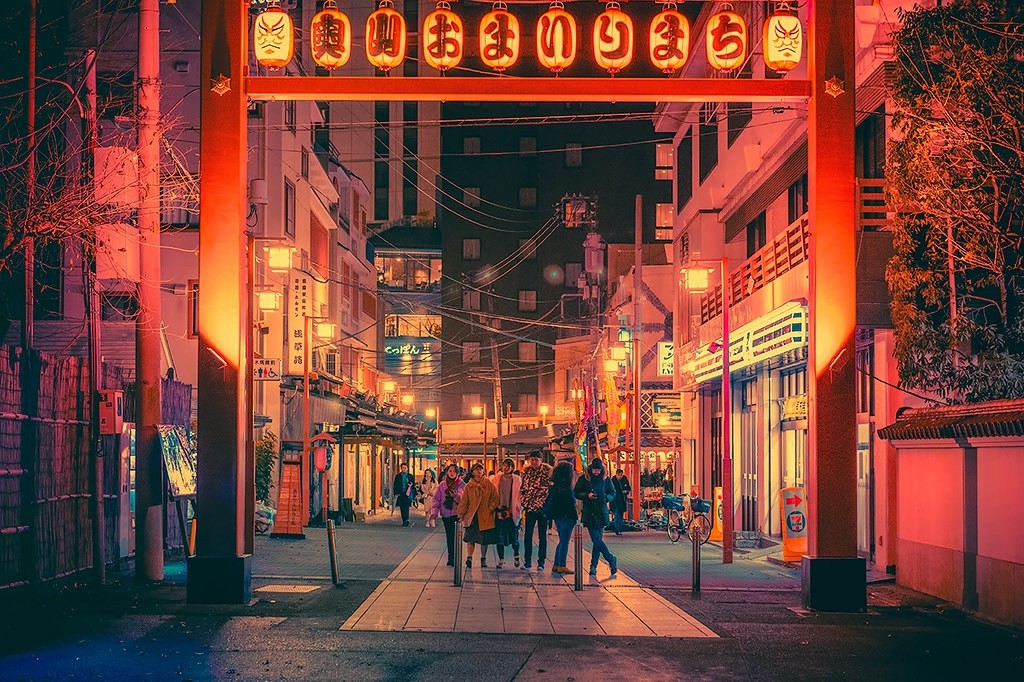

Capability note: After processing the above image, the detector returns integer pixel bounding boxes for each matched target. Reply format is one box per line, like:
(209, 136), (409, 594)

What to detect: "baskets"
(691, 498), (712, 513)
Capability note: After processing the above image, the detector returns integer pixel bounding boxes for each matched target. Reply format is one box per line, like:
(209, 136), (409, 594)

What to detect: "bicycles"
(666, 493), (712, 546)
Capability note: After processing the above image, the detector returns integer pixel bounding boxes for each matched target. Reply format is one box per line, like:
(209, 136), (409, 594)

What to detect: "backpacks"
(542, 482), (571, 519)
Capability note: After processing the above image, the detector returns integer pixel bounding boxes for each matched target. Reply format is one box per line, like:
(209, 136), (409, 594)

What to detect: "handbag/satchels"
(407, 474), (415, 501)
(497, 509), (511, 520)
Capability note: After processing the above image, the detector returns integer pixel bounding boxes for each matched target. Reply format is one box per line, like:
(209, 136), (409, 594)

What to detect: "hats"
(471, 462), (484, 473)
(590, 458), (602, 469)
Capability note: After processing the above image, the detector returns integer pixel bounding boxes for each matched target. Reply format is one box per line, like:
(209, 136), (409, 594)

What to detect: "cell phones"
(592, 494), (597, 497)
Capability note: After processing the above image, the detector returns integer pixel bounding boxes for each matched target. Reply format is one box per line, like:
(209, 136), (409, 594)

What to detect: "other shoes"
(557, 566), (574, 574)
(481, 557), (487, 567)
(520, 566), (529, 570)
(496, 560), (506, 568)
(610, 556), (617, 575)
(425, 521), (430, 528)
(552, 565), (558, 572)
(537, 566), (543, 571)
(466, 557), (472, 568)
(447, 560), (453, 566)
(431, 520), (435, 528)
(402, 520), (410, 527)
(590, 565), (596, 575)
(514, 556), (520, 567)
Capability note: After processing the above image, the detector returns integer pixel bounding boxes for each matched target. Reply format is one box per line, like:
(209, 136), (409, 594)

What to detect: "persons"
(609, 469), (631, 535)
(489, 470), (520, 478)
(457, 462), (498, 567)
(459, 467), (474, 483)
(520, 451), (555, 571)
(492, 458), (522, 568)
(548, 462), (578, 574)
(573, 459), (617, 575)
(393, 463), (466, 565)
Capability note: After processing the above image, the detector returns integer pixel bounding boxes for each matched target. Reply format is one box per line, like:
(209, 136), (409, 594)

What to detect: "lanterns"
(763, 12), (802, 72)
(366, 8), (406, 70)
(649, 8), (689, 72)
(480, 10), (520, 70)
(254, 8), (294, 71)
(310, 9), (351, 70)
(594, 9), (633, 71)
(315, 446), (331, 472)
(423, 9), (463, 70)
(536, 8), (577, 71)
(706, 10), (745, 72)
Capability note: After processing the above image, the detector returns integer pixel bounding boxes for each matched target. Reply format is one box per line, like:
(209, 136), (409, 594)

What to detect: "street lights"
(374, 376), (398, 510)
(680, 255), (733, 564)
(426, 407), (440, 476)
(303, 314), (341, 528)
(539, 405), (549, 426)
(612, 338), (642, 521)
(471, 404), (488, 476)
